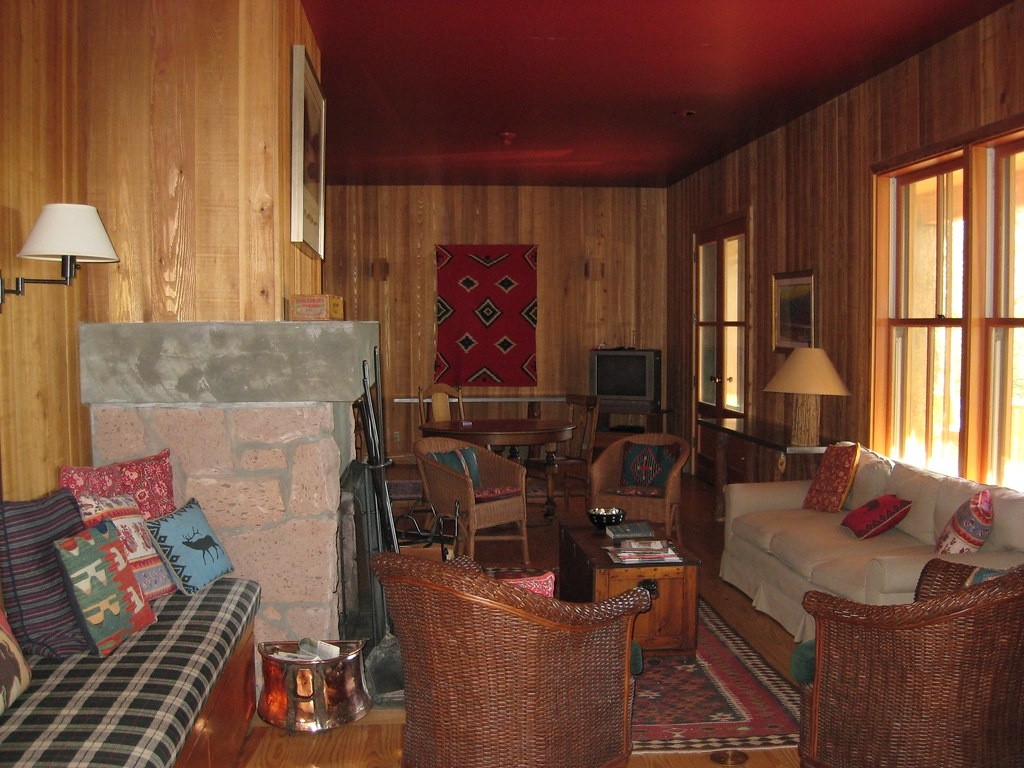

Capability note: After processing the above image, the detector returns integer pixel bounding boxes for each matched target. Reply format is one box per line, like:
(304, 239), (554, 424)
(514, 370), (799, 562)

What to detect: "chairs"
(586, 427), (693, 537)
(412, 435), (536, 566)
(526, 392), (603, 510)
(364, 548), (653, 768)
(788, 558), (1024, 768)
(414, 382), (469, 421)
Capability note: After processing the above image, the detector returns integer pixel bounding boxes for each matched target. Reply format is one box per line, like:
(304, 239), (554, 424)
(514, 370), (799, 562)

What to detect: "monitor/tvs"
(589, 348), (662, 412)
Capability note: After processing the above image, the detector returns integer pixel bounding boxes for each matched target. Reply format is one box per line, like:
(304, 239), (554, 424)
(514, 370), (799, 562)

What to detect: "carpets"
(387, 468), (586, 507)
(480, 559), (805, 756)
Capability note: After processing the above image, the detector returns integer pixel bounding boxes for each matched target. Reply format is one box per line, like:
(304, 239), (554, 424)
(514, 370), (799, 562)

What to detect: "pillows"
(426, 448), (481, 489)
(0, 486), (91, 660)
(802, 441), (861, 511)
(56, 446), (176, 520)
(51, 514), (159, 661)
(619, 440), (679, 489)
(933, 486), (991, 554)
(75, 490), (178, 601)
(0, 605), (34, 719)
(839, 492), (913, 538)
(144, 495), (236, 600)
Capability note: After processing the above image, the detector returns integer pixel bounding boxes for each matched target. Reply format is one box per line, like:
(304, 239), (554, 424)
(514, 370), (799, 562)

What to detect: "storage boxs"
(286, 292), (346, 321)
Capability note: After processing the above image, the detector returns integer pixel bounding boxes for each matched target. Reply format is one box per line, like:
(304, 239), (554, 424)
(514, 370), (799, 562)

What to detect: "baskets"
(589, 509), (626, 530)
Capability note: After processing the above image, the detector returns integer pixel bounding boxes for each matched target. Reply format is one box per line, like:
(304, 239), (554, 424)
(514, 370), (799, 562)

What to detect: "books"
(606, 536), (684, 563)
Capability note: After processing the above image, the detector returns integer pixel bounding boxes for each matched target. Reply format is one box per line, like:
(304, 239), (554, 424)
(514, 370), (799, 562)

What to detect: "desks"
(417, 413), (582, 525)
(694, 412), (852, 525)
(589, 402), (673, 468)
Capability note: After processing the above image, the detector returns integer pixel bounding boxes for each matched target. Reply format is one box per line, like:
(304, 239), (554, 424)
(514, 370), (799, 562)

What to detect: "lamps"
(0, 201), (122, 314)
(758, 342), (850, 445)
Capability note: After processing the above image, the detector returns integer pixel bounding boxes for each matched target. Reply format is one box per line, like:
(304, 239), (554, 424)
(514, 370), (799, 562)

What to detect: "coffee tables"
(554, 513), (703, 661)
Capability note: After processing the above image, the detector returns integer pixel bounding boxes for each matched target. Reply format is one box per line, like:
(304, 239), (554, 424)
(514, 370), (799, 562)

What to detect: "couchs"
(0, 446), (267, 768)
(720, 438), (1024, 643)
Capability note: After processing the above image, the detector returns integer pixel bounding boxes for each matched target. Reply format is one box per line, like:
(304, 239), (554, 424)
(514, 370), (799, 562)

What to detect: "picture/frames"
(772, 268), (816, 353)
(289, 39), (330, 262)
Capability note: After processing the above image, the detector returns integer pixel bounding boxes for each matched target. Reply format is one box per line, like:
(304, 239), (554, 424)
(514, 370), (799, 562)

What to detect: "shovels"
(361, 466), (405, 694)
